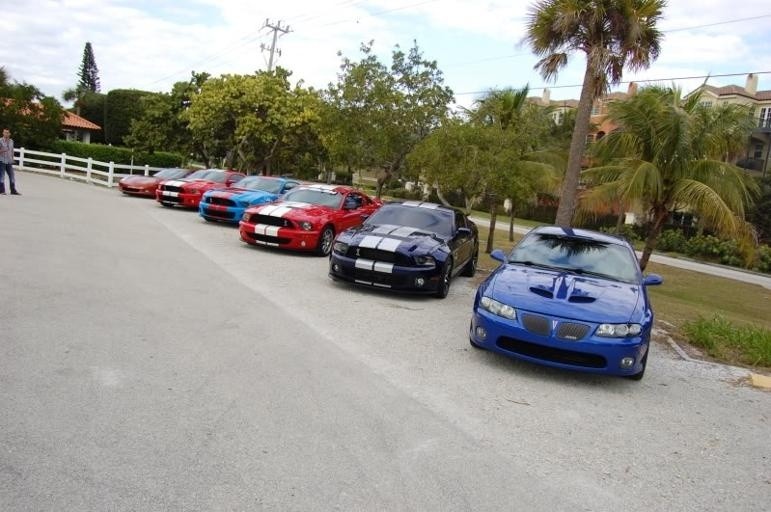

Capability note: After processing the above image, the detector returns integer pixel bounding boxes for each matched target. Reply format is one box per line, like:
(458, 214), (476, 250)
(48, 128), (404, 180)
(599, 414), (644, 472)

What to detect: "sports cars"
(468, 224), (662, 381)
(118, 168), (380, 254)
(328, 196), (479, 299)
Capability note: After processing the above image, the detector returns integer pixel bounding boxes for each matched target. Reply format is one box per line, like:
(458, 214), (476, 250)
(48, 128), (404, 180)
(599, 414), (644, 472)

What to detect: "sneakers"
(0, 189), (5, 194)
(11, 190), (22, 195)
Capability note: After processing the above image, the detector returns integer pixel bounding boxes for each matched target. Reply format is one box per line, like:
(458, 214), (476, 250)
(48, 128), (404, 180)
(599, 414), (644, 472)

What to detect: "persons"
(1, 127), (21, 195)
